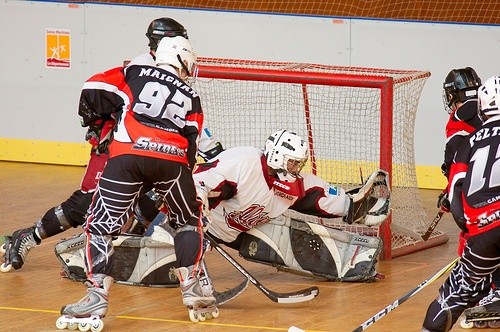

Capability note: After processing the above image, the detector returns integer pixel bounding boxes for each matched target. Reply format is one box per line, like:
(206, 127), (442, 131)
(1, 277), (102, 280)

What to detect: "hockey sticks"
(390, 208), (444, 243)
(198, 260), (251, 307)
(287, 257), (461, 332)
(84, 131), (320, 304)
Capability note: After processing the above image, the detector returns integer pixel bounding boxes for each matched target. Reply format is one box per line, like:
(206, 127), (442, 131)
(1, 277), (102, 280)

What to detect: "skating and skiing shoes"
(0, 224), (42, 272)
(172, 265), (221, 323)
(56, 275), (114, 332)
(460, 287), (500, 329)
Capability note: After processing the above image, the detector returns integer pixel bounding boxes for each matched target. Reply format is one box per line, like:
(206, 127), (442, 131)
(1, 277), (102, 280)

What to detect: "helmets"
(442, 66), (483, 115)
(145, 18), (189, 52)
(155, 36), (199, 86)
(264, 128), (309, 184)
(478, 76), (500, 127)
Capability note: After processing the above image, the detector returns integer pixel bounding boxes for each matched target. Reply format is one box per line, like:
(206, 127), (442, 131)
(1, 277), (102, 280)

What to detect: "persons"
(56, 35), (217, 331)
(55, 128), (390, 287)
(0, 16), (190, 274)
(421, 66), (500, 332)
